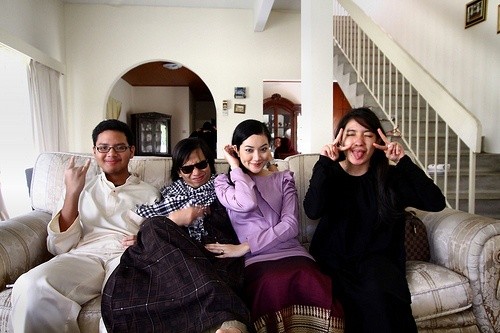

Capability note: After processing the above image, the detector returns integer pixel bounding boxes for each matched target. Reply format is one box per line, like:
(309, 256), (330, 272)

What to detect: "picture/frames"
(234, 104), (245, 113)
(465, 0), (486, 28)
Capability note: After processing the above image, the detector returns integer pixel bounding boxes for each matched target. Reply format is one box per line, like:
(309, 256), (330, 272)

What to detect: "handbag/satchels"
(406, 211), (430, 262)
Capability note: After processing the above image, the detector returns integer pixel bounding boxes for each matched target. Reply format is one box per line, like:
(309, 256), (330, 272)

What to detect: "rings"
(221, 249), (224, 254)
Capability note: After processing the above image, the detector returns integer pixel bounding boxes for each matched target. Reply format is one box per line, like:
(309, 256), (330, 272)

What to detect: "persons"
(6, 116), (164, 333)
(187, 122), (217, 160)
(273, 136), (294, 159)
(121, 136), (253, 332)
(304, 107), (446, 333)
(204, 119), (347, 332)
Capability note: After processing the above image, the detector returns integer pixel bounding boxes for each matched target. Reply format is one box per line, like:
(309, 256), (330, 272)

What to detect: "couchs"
(0, 151), (500, 333)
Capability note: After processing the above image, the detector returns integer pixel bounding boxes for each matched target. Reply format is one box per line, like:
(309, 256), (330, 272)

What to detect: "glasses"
(95, 145), (131, 153)
(178, 160), (210, 174)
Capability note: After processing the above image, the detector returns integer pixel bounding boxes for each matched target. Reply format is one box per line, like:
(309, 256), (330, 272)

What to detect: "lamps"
(162, 62), (180, 70)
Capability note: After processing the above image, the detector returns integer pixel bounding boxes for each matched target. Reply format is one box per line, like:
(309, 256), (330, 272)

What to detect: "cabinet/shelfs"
(130, 112), (172, 157)
(263, 93), (301, 152)
